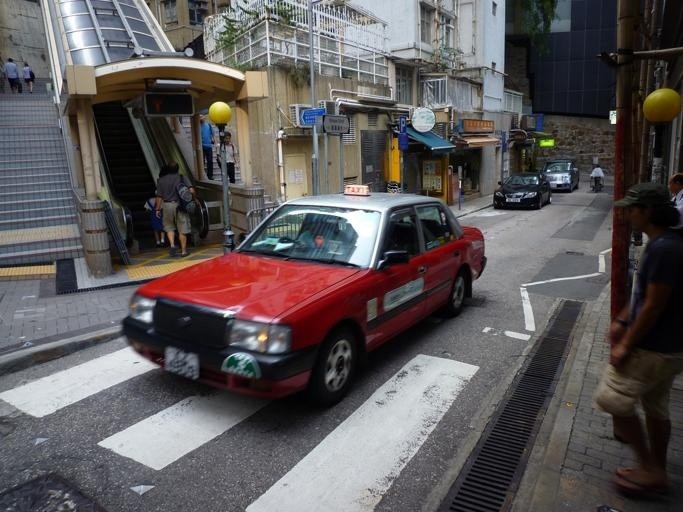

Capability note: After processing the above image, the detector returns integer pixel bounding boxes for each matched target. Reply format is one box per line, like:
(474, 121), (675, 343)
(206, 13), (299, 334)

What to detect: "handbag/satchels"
(176, 183), (196, 216)
(29, 70), (35, 79)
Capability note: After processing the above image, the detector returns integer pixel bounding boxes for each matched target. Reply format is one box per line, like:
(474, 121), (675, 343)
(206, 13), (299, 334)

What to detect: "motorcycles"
(590, 176), (604, 193)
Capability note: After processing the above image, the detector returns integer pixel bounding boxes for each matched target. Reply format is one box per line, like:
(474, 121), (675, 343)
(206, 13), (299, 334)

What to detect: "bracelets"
(156, 210), (161, 211)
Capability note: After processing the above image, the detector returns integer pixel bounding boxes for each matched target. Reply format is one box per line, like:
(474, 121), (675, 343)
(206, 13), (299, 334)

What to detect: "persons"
(200, 114), (216, 180)
(216, 131), (237, 183)
(155, 161), (199, 257)
(593, 183), (683, 495)
(143, 190), (166, 248)
(2, 58), (19, 93)
(22, 64), (35, 93)
(590, 164), (605, 188)
(668, 172), (683, 230)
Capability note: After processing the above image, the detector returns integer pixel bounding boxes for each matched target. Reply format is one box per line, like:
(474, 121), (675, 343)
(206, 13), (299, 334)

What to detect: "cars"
(493, 172), (551, 210)
(540, 158), (578, 192)
(122, 184), (488, 407)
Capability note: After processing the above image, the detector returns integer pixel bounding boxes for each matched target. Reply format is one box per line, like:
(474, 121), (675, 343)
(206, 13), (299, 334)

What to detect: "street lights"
(208, 101), (235, 254)
(642, 87), (681, 187)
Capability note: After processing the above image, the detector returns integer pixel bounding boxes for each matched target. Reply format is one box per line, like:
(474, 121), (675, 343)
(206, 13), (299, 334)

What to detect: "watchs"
(616, 319), (628, 327)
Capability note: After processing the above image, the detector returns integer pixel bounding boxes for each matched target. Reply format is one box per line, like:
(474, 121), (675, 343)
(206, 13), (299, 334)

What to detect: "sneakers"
(155, 243), (164, 246)
(182, 252), (190, 257)
(170, 247), (176, 256)
(614, 467), (667, 496)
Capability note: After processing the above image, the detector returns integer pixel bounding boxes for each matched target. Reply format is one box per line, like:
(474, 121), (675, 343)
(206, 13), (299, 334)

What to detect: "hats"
(613, 182), (668, 208)
